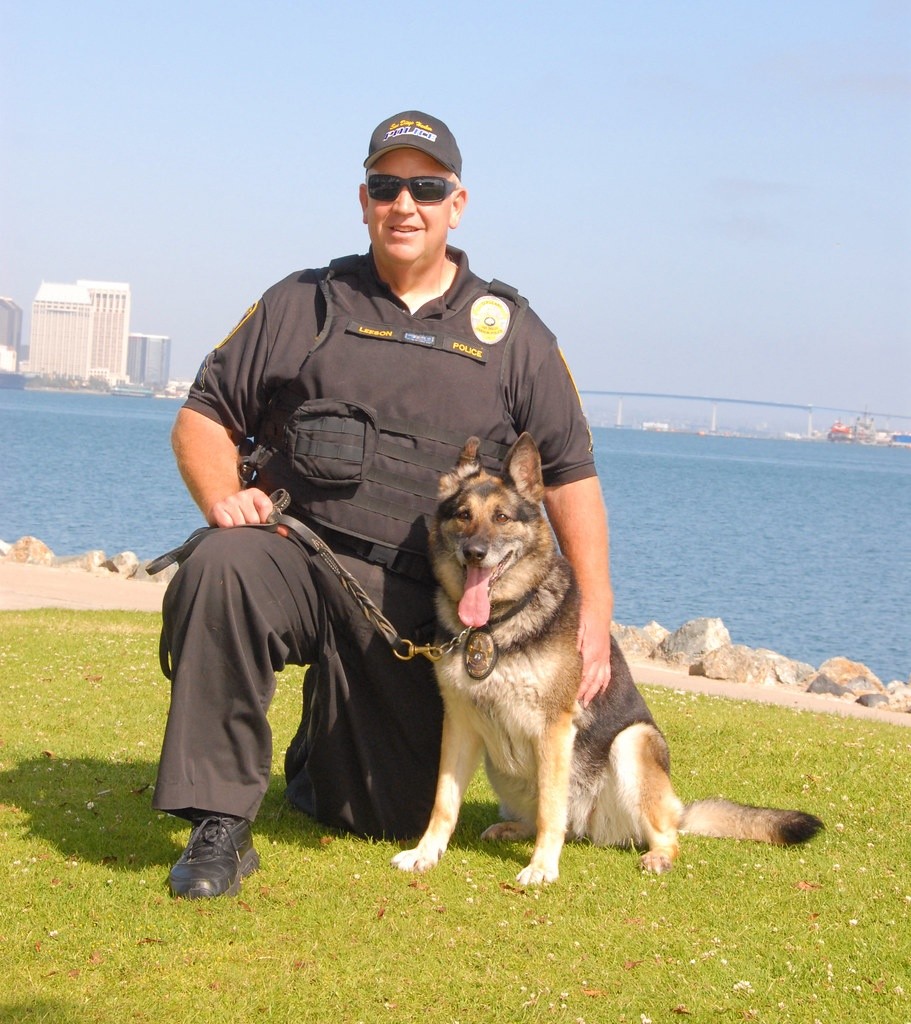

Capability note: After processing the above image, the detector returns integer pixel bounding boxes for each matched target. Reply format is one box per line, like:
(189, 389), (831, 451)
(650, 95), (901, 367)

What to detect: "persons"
(154, 111), (613, 899)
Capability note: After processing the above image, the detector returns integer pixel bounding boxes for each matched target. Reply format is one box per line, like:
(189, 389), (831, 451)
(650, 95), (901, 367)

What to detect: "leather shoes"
(169, 810), (259, 902)
(285, 664), (322, 787)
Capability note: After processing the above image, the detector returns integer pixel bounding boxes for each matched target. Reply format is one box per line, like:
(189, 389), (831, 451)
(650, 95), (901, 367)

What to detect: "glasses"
(365, 174), (456, 203)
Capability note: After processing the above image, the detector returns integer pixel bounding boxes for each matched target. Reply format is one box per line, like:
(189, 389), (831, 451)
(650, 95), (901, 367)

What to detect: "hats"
(363, 110), (462, 182)
